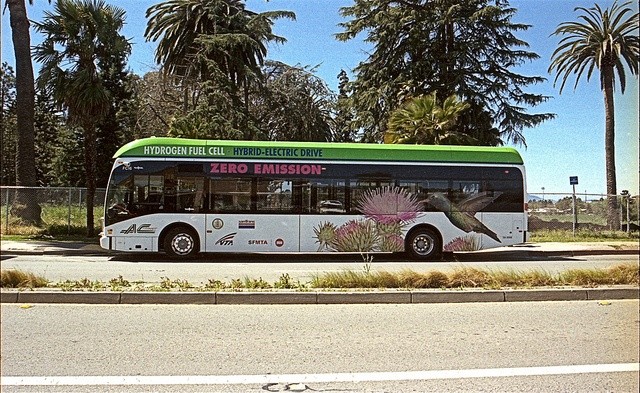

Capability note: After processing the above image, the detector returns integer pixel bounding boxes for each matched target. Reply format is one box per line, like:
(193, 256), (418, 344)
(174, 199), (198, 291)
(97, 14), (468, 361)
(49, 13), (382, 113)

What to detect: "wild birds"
(420, 190), (503, 243)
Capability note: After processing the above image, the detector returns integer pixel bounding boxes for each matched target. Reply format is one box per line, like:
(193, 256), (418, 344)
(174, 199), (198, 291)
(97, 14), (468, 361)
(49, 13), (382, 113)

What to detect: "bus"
(98, 136), (528, 261)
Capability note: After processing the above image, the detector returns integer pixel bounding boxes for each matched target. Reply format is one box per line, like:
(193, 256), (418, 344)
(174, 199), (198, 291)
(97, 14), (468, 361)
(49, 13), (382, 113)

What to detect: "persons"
(138, 186), (162, 213)
